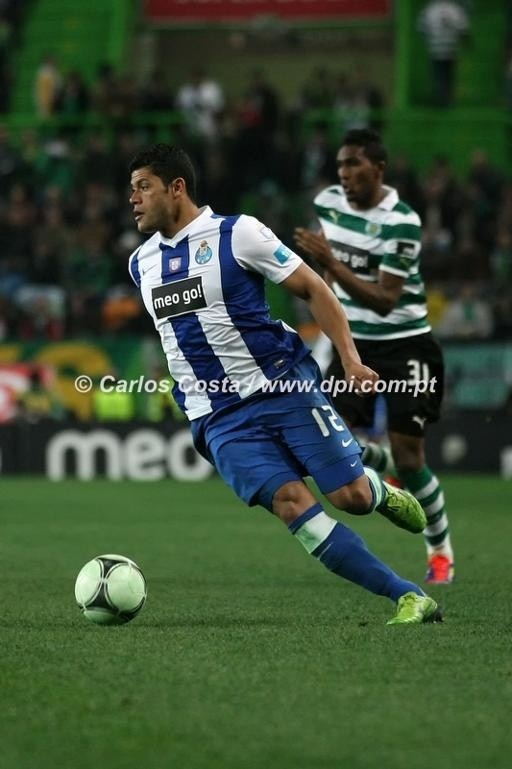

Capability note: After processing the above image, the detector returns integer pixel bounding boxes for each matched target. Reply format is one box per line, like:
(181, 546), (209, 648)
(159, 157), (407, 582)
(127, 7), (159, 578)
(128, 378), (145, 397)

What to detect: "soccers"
(74, 553), (147, 625)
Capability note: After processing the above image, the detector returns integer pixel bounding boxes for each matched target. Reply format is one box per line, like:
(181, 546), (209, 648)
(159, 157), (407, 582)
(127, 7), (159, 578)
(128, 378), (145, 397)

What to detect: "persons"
(124, 140), (445, 630)
(290, 128), (458, 589)
(2, 1), (509, 341)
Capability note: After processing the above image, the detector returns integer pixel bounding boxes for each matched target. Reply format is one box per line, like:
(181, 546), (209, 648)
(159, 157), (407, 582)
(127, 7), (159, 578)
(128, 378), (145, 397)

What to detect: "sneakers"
(375, 480), (428, 534)
(422, 552), (455, 585)
(386, 591), (442, 626)
